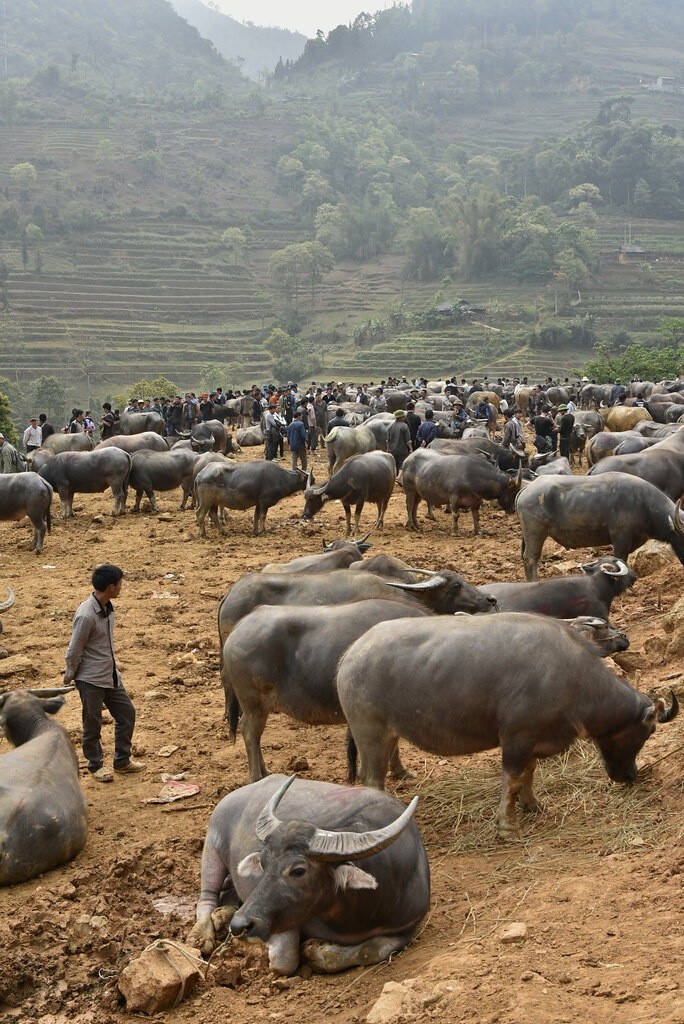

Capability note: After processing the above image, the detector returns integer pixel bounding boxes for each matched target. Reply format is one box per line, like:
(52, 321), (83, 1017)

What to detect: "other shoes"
(304, 446), (326, 455)
(272, 456), (285, 463)
(395, 476), (403, 487)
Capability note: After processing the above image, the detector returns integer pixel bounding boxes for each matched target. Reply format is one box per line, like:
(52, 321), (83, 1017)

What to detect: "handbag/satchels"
(533, 435), (546, 450)
(546, 400), (553, 410)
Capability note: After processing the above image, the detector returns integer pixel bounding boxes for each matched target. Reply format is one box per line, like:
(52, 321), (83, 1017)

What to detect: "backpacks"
(14, 451), (31, 472)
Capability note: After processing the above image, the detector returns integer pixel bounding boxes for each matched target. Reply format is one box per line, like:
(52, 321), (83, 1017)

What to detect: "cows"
(0, 472), (53, 554)
(184, 770), (431, 976)
(513, 471), (684, 584)
(0, 685), (89, 889)
(213, 532), (638, 784)
(20, 376), (683, 538)
(336, 611), (678, 841)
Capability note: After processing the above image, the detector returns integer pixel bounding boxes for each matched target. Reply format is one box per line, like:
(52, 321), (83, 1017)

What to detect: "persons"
(0, 431), (26, 474)
(63, 564), (147, 782)
(22, 372), (682, 459)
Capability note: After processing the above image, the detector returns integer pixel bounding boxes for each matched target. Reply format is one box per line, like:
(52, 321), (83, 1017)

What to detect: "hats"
(0, 433), (4, 439)
(453, 400), (464, 407)
(551, 405), (558, 413)
(581, 377), (589, 381)
(203, 393), (207, 397)
(29, 415), (37, 422)
(558, 404), (569, 411)
(127, 399), (132, 404)
(614, 378), (621, 385)
(394, 409), (408, 418)
(409, 387), (418, 393)
(138, 399), (144, 404)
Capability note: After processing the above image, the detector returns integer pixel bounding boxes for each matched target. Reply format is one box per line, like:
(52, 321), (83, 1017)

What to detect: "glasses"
(31, 420), (36, 421)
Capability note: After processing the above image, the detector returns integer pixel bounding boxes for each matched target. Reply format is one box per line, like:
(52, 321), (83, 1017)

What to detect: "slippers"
(92, 767), (114, 781)
(114, 760), (147, 773)
